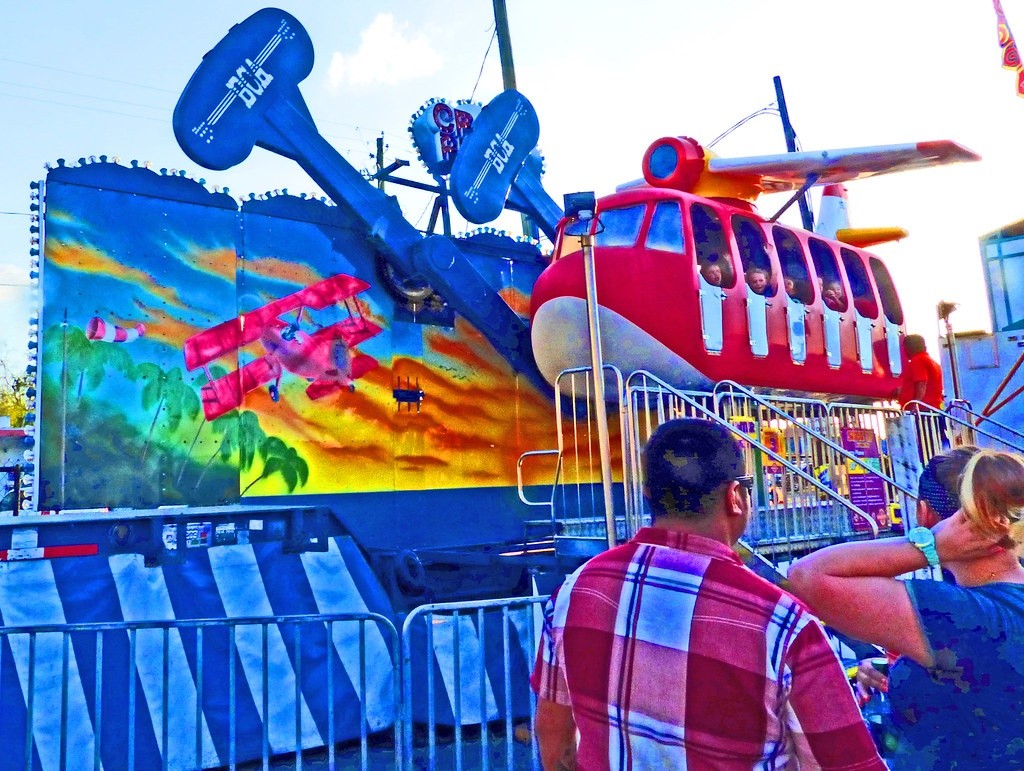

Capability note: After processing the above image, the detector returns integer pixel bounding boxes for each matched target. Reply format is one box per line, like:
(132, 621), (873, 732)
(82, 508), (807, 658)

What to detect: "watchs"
(908, 525), (939, 566)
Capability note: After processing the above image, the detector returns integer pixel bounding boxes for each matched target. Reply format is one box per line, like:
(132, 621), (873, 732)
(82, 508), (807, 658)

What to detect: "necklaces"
(978, 565), (1020, 584)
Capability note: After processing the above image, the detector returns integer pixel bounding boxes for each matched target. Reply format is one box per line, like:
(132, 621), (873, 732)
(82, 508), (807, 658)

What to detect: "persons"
(897, 335), (951, 462)
(787, 445), (1024, 771)
(529, 418), (889, 771)
(705, 260), (845, 313)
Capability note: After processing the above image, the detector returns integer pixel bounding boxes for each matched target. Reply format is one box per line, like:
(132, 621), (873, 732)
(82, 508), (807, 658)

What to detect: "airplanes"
(526, 134), (981, 412)
